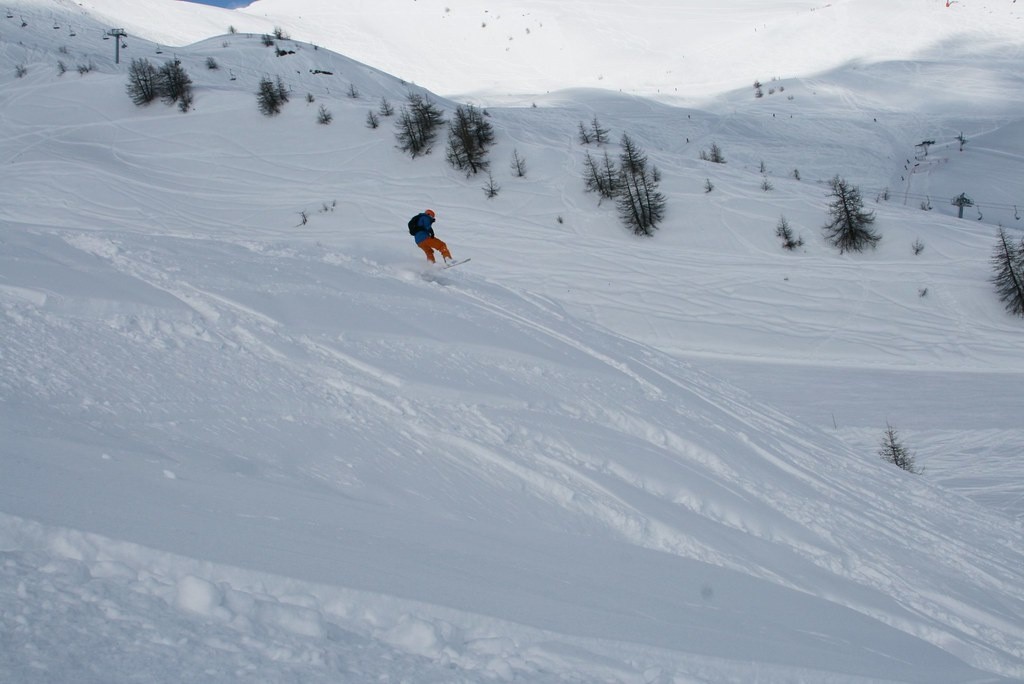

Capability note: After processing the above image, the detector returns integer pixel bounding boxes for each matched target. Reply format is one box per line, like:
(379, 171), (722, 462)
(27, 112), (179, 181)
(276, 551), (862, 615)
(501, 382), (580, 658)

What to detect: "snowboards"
(443, 256), (472, 269)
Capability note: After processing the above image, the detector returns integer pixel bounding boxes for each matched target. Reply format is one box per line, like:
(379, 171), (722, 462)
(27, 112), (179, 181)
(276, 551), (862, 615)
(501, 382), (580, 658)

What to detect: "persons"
(413, 209), (456, 266)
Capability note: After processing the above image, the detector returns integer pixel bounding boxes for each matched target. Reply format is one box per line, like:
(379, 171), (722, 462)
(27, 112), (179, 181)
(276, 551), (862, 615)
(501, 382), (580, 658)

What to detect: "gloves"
(428, 228), (434, 239)
(430, 219), (435, 225)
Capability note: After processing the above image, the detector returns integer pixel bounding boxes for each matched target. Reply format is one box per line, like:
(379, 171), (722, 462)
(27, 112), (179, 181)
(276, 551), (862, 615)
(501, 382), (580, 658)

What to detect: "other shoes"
(445, 257), (455, 265)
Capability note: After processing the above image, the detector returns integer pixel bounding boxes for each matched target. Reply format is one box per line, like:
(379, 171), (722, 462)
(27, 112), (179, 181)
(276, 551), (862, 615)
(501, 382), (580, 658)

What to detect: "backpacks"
(408, 213), (428, 236)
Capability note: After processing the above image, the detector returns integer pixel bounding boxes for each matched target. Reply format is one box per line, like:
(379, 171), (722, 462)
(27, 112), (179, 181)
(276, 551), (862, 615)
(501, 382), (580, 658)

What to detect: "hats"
(425, 209), (435, 217)
